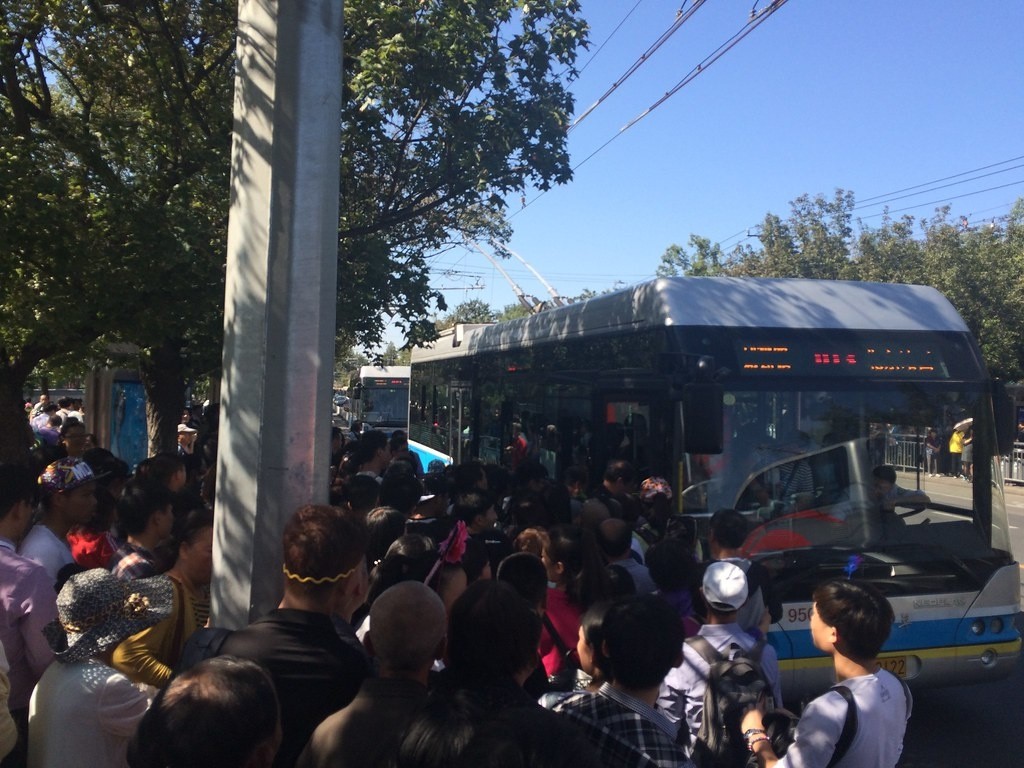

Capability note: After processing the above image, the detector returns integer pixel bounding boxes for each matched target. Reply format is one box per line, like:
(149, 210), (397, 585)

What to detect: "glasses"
(63, 432), (87, 441)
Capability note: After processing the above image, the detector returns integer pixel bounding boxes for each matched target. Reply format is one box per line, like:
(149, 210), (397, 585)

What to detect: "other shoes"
(929, 472), (973, 482)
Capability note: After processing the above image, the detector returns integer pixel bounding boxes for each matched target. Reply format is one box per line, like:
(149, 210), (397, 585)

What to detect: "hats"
(639, 476), (672, 503)
(701, 562), (748, 611)
(41, 568), (174, 664)
(37, 456), (113, 497)
(177, 424), (197, 433)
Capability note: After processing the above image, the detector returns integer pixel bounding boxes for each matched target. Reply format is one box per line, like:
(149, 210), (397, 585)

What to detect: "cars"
(681, 437), (897, 556)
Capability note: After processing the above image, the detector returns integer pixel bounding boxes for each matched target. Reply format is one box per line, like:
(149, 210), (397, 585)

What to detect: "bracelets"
(747, 736), (772, 752)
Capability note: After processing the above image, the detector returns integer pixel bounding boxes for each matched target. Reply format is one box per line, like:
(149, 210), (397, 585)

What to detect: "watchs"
(743, 729), (767, 741)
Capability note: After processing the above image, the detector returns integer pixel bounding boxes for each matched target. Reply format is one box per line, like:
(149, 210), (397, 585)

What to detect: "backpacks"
(684, 635), (775, 768)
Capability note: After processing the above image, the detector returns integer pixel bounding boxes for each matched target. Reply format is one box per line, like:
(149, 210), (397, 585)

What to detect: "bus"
(408, 273), (1023, 688)
(333, 386), (349, 421)
(348, 364), (411, 441)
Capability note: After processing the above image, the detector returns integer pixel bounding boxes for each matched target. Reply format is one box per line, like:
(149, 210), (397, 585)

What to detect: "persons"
(1017, 419), (1024, 442)
(739, 582), (913, 768)
(212, 425), (633, 768)
(438, 414), (931, 529)
(884, 423), (974, 483)
(0, 392), (283, 768)
(581, 459), (783, 768)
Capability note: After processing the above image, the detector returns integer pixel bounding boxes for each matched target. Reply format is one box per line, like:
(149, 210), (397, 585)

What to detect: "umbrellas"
(954, 417), (973, 431)
